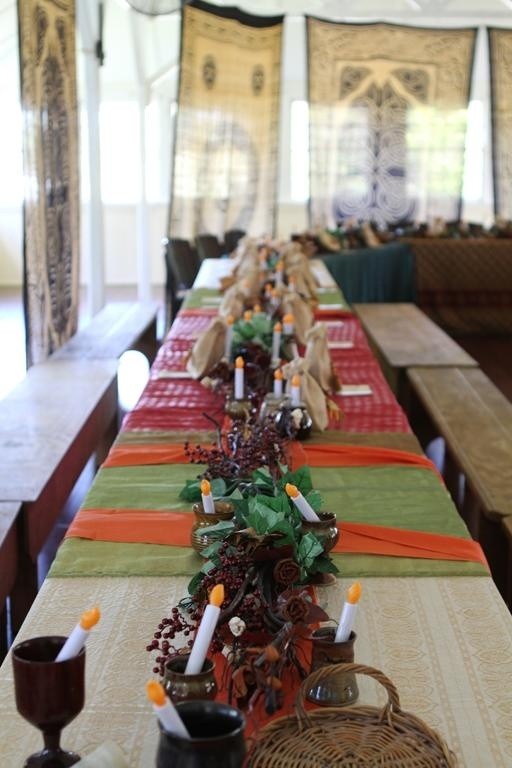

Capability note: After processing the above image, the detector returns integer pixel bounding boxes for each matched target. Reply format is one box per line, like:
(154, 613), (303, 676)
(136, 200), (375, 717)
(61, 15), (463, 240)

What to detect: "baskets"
(246, 662), (460, 768)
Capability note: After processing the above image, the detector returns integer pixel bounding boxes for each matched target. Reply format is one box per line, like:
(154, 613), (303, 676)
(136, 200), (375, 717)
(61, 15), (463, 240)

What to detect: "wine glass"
(225, 394), (253, 430)
(296, 513), (340, 586)
(12, 637), (85, 765)
(156, 702), (248, 767)
(162, 659), (218, 705)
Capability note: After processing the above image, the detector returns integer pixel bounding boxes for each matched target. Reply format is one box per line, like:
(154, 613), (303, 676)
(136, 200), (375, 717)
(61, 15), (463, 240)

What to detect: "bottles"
(272, 402), (313, 440)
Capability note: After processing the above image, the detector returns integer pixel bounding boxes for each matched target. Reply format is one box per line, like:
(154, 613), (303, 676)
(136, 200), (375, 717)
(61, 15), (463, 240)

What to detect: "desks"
(316, 235), (511, 336)
(2, 258), (512, 768)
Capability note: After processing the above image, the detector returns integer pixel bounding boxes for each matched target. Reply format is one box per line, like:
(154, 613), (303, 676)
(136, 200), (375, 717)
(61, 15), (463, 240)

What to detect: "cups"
(305, 625), (360, 707)
(191, 501), (236, 560)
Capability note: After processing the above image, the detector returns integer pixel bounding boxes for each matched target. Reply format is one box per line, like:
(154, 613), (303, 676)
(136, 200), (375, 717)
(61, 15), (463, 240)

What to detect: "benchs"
(403, 363), (512, 542)
(0, 300), (165, 651)
(353, 295), (480, 393)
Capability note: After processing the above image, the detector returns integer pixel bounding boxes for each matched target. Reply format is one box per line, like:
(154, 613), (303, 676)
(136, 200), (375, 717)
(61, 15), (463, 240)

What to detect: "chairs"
(165, 230), (246, 324)
